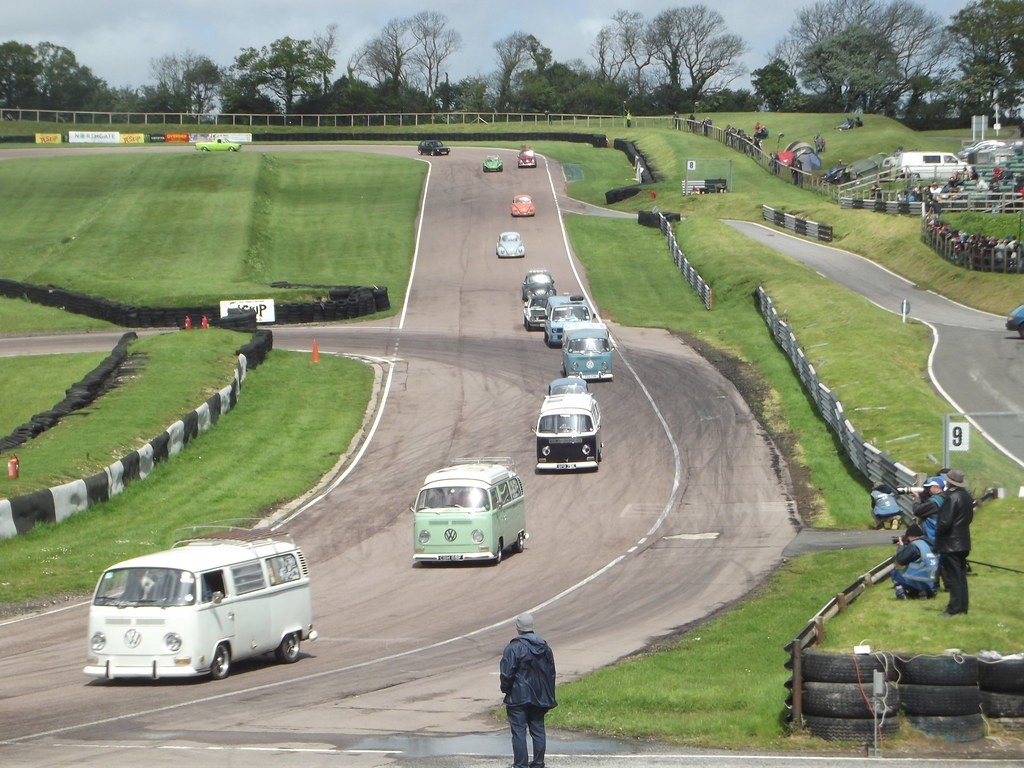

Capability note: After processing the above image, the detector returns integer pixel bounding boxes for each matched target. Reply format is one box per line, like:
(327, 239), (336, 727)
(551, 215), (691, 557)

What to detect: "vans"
(560, 322), (615, 380)
(898, 152), (972, 181)
(409, 456), (529, 563)
(545, 295), (596, 347)
(531, 393), (603, 470)
(82, 518), (318, 680)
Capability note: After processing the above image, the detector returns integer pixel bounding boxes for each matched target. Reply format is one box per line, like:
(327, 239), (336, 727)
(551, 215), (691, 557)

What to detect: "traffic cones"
(311, 338), (321, 363)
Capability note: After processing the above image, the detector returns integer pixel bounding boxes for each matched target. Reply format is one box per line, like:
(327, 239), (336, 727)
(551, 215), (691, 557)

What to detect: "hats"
(906, 524), (923, 536)
(944, 469), (968, 488)
(923, 477), (946, 488)
(516, 613), (534, 632)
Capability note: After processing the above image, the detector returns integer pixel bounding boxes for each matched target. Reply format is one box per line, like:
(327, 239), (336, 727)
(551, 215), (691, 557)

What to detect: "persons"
(625, 109), (631, 127)
(870, 481), (903, 530)
(909, 478), (949, 593)
(940, 166), (1024, 213)
(673, 112), (680, 129)
(935, 470), (972, 615)
(770, 152), (801, 184)
(545, 109), (552, 125)
(814, 135), (826, 152)
(686, 115), (712, 136)
(890, 524), (938, 600)
(500, 614), (557, 768)
(841, 117), (862, 129)
(896, 181), (942, 201)
(871, 185), (881, 197)
(753, 122), (768, 138)
(724, 124), (760, 155)
(926, 199), (1024, 273)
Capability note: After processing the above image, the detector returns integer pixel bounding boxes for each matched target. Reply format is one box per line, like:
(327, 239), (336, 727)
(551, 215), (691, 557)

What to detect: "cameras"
(897, 486), (929, 496)
(892, 535), (909, 545)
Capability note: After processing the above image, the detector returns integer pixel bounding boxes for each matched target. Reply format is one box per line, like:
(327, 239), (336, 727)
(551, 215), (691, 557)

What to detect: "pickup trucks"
(195, 138), (242, 151)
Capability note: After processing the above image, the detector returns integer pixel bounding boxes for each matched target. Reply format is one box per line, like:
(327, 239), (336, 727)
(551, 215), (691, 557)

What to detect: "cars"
(417, 140), (450, 156)
(517, 151), (537, 169)
(957, 139), (1024, 157)
(1005, 304), (1024, 338)
(522, 270), (557, 331)
(482, 155), (503, 172)
(512, 194), (536, 217)
(548, 378), (588, 396)
(496, 232), (525, 258)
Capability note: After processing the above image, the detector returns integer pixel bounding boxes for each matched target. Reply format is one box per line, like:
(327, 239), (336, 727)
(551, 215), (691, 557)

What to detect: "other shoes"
(890, 519), (899, 530)
(918, 590), (929, 601)
(897, 592), (909, 601)
(878, 524), (885, 531)
(966, 563), (971, 572)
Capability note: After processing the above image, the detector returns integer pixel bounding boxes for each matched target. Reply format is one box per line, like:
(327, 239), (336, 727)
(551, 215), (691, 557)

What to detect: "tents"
(796, 152), (821, 171)
